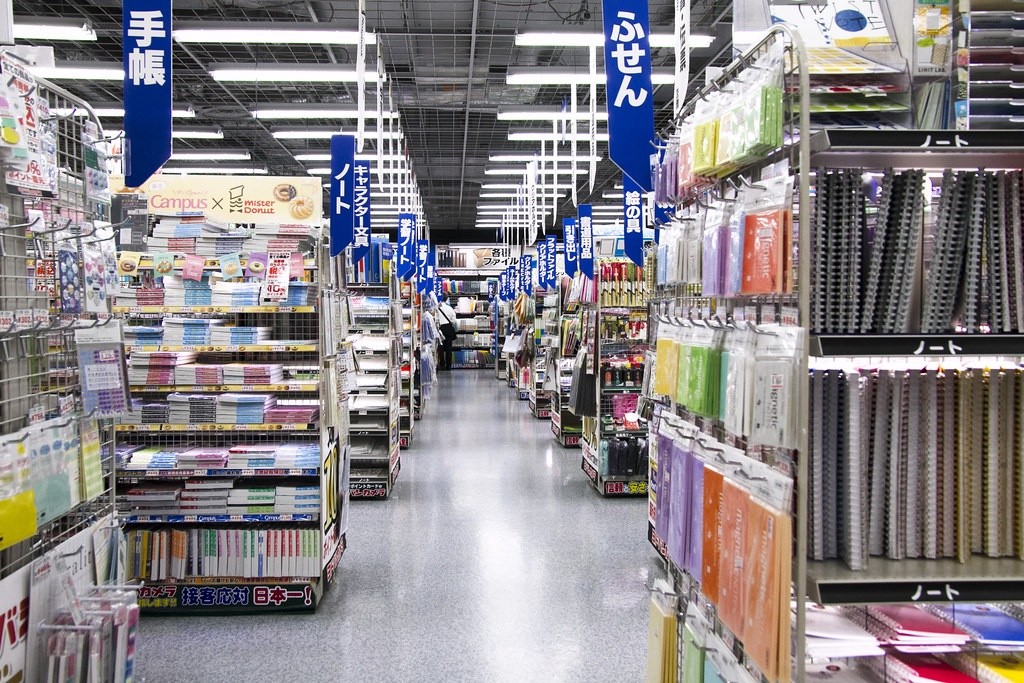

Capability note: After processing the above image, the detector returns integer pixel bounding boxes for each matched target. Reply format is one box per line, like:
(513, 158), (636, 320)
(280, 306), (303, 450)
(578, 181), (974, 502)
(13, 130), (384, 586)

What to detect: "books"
(789, 162), (1024, 683)
(101, 217), (409, 581)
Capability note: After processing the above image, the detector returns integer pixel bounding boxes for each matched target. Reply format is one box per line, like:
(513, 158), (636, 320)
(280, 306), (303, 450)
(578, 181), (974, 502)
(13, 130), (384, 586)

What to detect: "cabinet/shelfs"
(0, 51), (652, 683)
(653, 23), (1024, 683)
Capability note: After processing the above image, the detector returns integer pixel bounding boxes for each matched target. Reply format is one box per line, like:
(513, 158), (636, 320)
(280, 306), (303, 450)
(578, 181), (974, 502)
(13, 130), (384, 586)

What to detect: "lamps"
(11, 15), (427, 229)
(472, 29), (716, 229)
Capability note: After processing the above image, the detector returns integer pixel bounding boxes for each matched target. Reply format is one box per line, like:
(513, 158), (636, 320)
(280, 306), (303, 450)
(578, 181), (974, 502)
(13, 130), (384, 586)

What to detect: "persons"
(437, 292), (456, 371)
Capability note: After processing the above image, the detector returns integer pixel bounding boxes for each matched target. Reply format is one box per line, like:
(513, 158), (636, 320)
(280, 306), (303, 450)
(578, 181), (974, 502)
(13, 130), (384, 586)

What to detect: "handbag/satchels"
(449, 323), (457, 340)
(502, 330), (522, 353)
